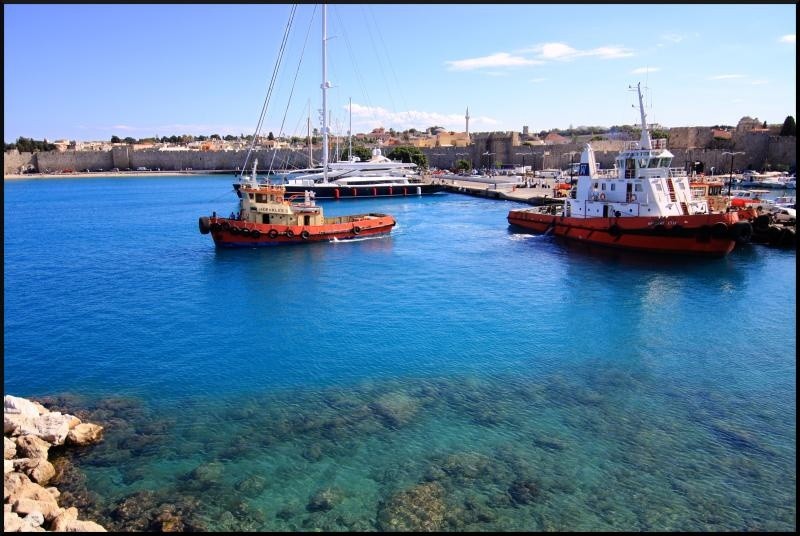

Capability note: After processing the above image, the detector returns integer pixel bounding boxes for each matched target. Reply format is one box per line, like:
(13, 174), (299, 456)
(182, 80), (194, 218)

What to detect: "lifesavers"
(600, 193), (605, 200)
(609, 225), (619, 236)
(632, 194), (637, 201)
(198, 216), (209, 234)
(734, 221), (753, 242)
(301, 230), (310, 239)
(215, 222), (260, 239)
(713, 222), (728, 238)
(393, 221), (395, 226)
(276, 196), (282, 203)
(286, 229), (294, 237)
(754, 215), (771, 232)
(352, 226), (361, 235)
(269, 228), (278, 239)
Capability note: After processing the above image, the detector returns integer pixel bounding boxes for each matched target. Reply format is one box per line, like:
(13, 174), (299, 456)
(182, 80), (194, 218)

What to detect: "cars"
(92, 167), (160, 172)
(730, 191), (763, 209)
(767, 206), (796, 223)
(80, 168), (90, 172)
(62, 168), (72, 173)
(432, 167), (571, 183)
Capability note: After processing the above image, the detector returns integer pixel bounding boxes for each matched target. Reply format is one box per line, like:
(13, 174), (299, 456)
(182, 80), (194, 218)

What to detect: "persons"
(229, 212), (240, 220)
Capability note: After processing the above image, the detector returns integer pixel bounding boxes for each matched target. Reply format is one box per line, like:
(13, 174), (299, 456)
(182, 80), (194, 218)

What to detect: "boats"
(507, 81), (769, 256)
(200, 161), (396, 250)
(741, 172), (796, 189)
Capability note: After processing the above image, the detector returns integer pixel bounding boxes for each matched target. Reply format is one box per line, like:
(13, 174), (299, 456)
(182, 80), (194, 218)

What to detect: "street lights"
(515, 152), (541, 177)
(563, 152), (581, 188)
(721, 151), (746, 212)
(482, 152), (496, 170)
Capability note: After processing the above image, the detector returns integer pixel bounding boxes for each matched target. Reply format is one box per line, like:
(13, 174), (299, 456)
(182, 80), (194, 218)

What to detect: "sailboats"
(233, 4), (441, 201)
